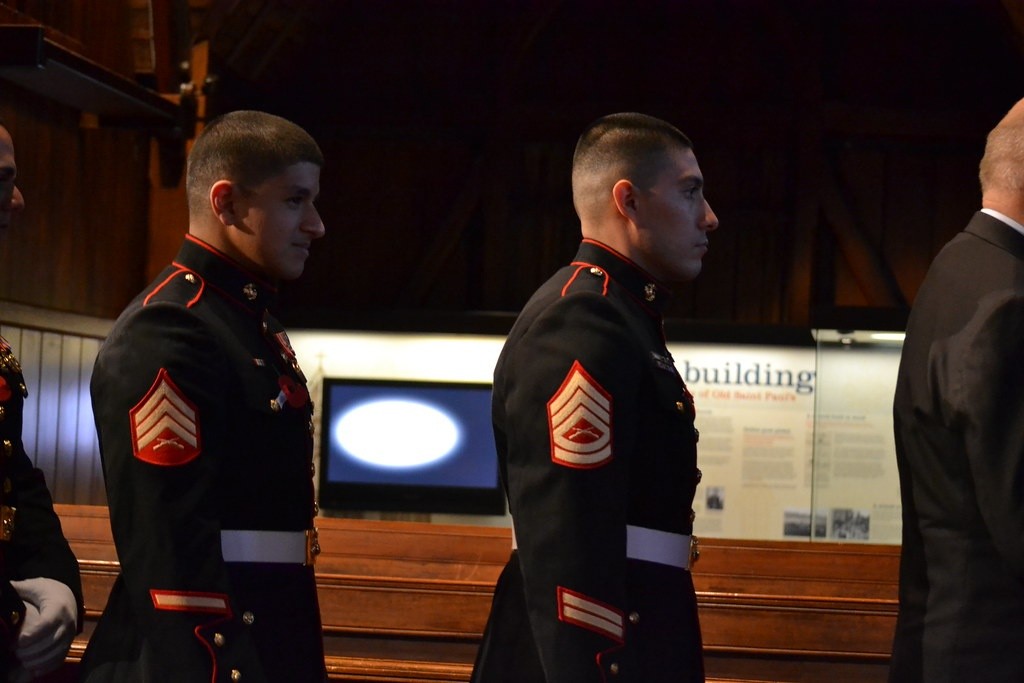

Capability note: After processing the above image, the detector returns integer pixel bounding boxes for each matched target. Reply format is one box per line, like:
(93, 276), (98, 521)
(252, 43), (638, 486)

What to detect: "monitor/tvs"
(316, 377), (508, 517)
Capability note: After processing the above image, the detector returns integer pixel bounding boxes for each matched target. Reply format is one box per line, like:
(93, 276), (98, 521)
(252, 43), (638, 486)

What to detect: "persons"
(470, 112), (722, 683)
(89, 109), (328, 683)
(889, 99), (1024, 683)
(0, 123), (87, 683)
